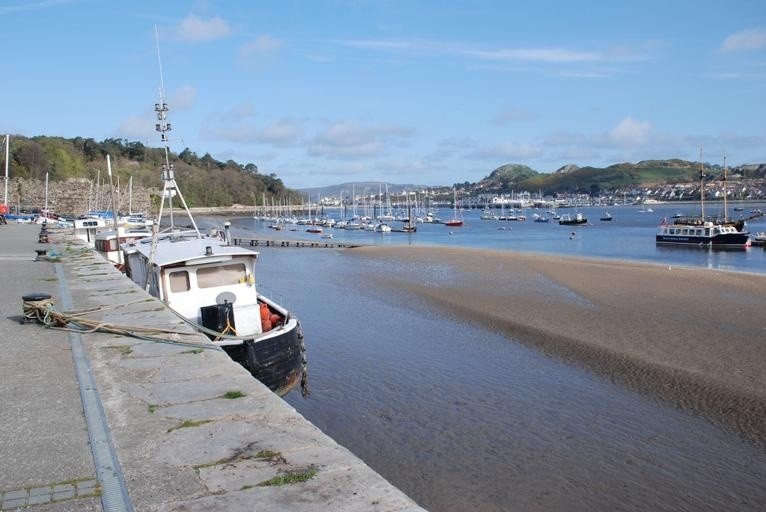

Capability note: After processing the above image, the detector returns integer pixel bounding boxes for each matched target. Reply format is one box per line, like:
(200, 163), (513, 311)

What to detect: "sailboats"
(315, 182), (442, 234)
(442, 187), (464, 227)
(0, 23), (316, 404)
(480, 189), (561, 223)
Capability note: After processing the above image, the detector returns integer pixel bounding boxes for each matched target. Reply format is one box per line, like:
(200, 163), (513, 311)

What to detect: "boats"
(655, 141), (766, 251)
(558, 212), (588, 226)
(638, 208), (654, 213)
(320, 234), (333, 239)
(600, 210), (614, 222)
(643, 199), (665, 206)
